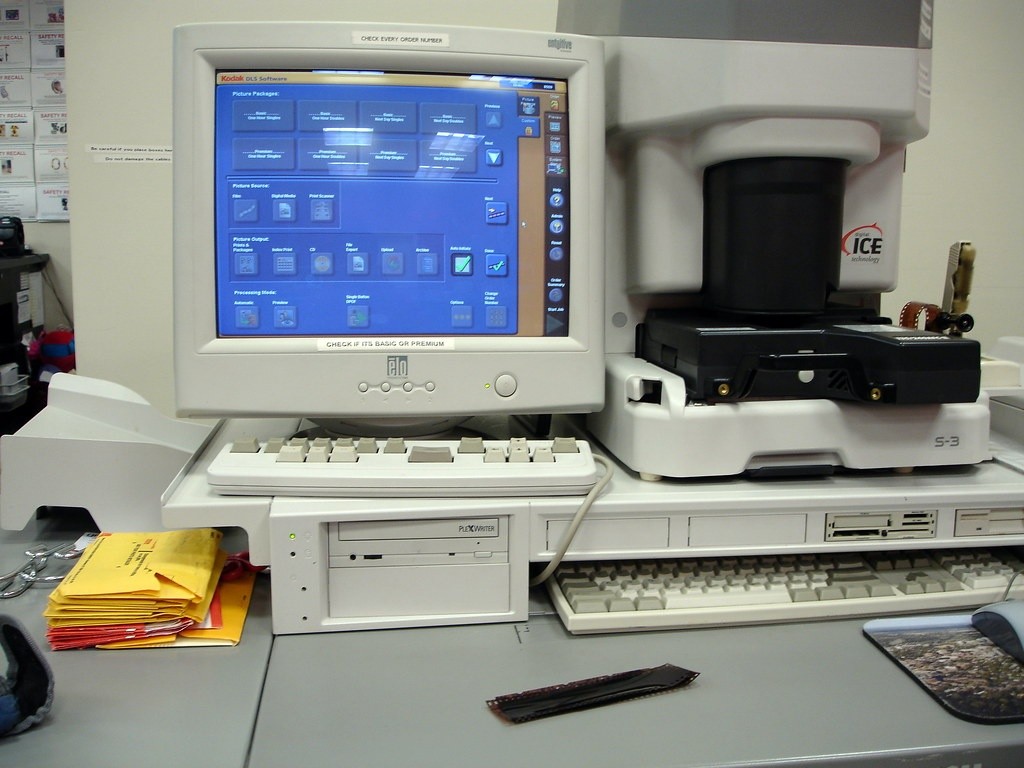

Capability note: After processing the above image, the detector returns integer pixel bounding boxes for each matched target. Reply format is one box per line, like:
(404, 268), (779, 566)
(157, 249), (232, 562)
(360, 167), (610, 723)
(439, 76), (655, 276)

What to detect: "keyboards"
(207, 436), (598, 499)
(543, 545), (1024, 636)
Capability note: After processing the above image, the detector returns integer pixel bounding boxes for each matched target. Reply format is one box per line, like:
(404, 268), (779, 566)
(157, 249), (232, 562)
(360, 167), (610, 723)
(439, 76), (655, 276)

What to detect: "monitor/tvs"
(172, 21), (607, 443)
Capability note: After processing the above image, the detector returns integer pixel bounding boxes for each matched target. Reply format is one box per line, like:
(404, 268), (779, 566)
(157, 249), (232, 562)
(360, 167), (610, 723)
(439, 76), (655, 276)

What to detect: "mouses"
(972, 598), (1024, 667)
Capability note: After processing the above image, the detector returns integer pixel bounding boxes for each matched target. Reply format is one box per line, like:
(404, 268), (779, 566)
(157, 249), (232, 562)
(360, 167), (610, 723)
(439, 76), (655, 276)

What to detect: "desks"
(1, 504), (272, 766)
(245, 506), (1024, 768)
